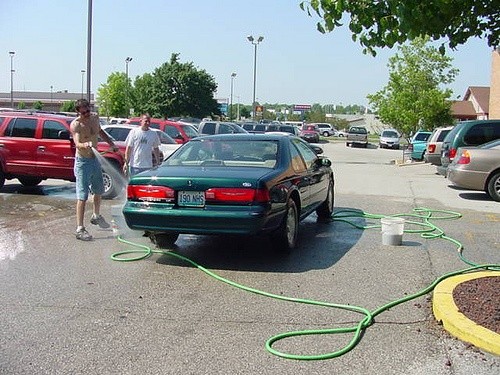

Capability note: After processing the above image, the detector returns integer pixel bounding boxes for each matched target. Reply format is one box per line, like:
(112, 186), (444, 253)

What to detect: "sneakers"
(90, 215), (110, 228)
(75, 227), (92, 241)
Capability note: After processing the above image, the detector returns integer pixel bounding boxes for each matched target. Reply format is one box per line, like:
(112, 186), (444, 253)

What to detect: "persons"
(123, 114), (161, 180)
(70, 99), (119, 241)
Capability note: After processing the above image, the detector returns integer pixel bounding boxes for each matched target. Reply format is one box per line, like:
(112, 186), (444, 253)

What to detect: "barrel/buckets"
(381, 217), (405, 246)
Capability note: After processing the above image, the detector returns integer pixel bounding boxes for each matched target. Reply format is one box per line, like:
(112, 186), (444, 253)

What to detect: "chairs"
(262, 154), (276, 162)
(215, 150), (234, 160)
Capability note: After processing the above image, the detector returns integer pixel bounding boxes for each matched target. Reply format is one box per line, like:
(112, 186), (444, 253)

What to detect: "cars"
(122, 129), (334, 256)
(42, 110), (344, 161)
(408, 130), (433, 162)
(446, 138), (500, 203)
(378, 129), (402, 150)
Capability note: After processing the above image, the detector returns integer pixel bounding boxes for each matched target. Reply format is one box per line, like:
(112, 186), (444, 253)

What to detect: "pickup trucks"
(344, 126), (370, 148)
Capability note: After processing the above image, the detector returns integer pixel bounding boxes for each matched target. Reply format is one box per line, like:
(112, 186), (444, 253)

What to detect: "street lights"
(247, 35), (264, 121)
(125, 57), (133, 89)
(230, 73), (237, 123)
(81, 69), (86, 98)
(8, 52), (15, 108)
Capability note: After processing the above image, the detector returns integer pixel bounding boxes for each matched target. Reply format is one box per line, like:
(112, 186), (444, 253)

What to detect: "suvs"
(434, 119), (500, 179)
(0, 111), (128, 200)
(423, 125), (455, 166)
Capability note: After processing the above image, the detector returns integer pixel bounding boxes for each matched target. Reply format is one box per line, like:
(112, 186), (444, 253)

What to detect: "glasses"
(79, 109), (90, 115)
